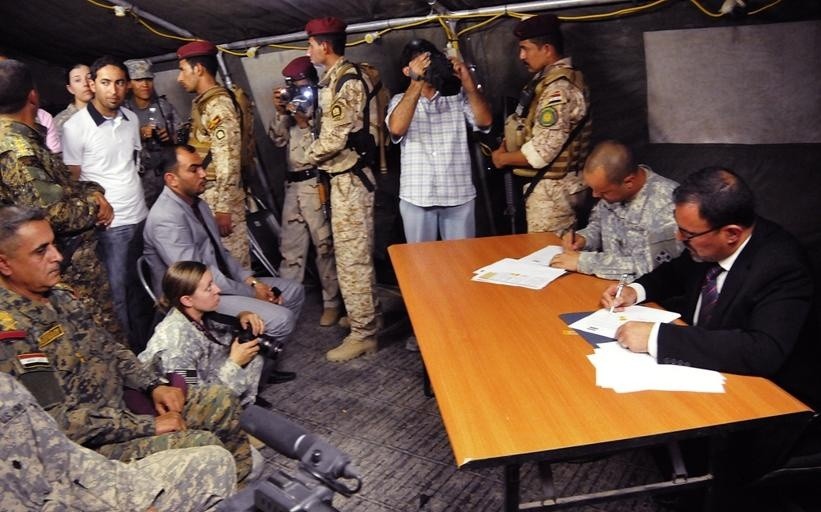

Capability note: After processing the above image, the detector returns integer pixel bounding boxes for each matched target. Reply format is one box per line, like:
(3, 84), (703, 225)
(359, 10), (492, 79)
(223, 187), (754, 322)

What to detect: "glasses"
(672, 228), (717, 241)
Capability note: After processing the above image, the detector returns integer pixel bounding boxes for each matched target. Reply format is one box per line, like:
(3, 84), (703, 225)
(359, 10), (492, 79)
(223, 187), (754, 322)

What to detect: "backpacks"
(332, 62), (391, 146)
(192, 85), (255, 170)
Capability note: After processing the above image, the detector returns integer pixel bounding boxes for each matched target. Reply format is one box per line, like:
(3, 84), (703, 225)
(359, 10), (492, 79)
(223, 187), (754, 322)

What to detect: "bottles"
(149, 108), (160, 144)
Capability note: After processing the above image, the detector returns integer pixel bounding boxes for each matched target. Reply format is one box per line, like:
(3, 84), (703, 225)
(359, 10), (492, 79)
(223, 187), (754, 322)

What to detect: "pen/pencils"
(610, 274), (627, 312)
(570, 231), (577, 245)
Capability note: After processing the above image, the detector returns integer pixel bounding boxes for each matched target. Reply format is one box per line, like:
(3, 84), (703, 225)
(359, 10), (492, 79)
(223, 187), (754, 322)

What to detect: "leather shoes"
(267, 368), (296, 384)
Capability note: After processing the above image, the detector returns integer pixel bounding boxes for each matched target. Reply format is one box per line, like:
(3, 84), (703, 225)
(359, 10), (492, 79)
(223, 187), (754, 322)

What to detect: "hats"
(514, 14), (559, 39)
(123, 58), (155, 80)
(304, 16), (344, 36)
(126, 371), (188, 414)
(176, 42), (219, 59)
(282, 56), (313, 80)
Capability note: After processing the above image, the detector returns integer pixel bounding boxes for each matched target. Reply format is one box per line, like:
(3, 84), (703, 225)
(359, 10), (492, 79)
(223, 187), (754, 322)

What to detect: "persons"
(489, 13), (594, 236)
(0, 35), (343, 512)
(549, 138), (683, 285)
(386, 37), (496, 354)
(598, 163), (820, 511)
(303, 16), (384, 365)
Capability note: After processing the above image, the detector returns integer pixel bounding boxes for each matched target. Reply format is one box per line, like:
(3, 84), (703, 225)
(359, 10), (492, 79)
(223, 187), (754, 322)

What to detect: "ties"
(698, 265), (726, 330)
(191, 204), (232, 279)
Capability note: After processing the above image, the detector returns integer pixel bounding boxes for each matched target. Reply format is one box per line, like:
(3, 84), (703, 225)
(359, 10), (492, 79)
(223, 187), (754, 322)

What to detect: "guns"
(309, 85), (331, 222)
(502, 169), (522, 234)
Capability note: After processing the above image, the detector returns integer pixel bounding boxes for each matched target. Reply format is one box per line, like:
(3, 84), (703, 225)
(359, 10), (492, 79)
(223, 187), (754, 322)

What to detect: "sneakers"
(406, 336), (418, 351)
(326, 335), (379, 362)
(319, 306), (339, 326)
(338, 317), (349, 329)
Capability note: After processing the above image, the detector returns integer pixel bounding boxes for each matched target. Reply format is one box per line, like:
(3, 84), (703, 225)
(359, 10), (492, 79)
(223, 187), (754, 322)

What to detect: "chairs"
(136, 254), (168, 319)
(244, 190), (281, 279)
(747, 409), (820, 491)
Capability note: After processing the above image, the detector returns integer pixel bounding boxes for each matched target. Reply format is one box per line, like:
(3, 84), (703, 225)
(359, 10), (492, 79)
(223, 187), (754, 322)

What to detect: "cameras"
(420, 50), (464, 99)
(233, 324), (284, 360)
(272, 76), (315, 118)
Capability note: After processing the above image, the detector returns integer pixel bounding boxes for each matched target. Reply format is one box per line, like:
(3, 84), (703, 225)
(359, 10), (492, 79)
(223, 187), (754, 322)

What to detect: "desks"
(387, 230), (817, 512)
